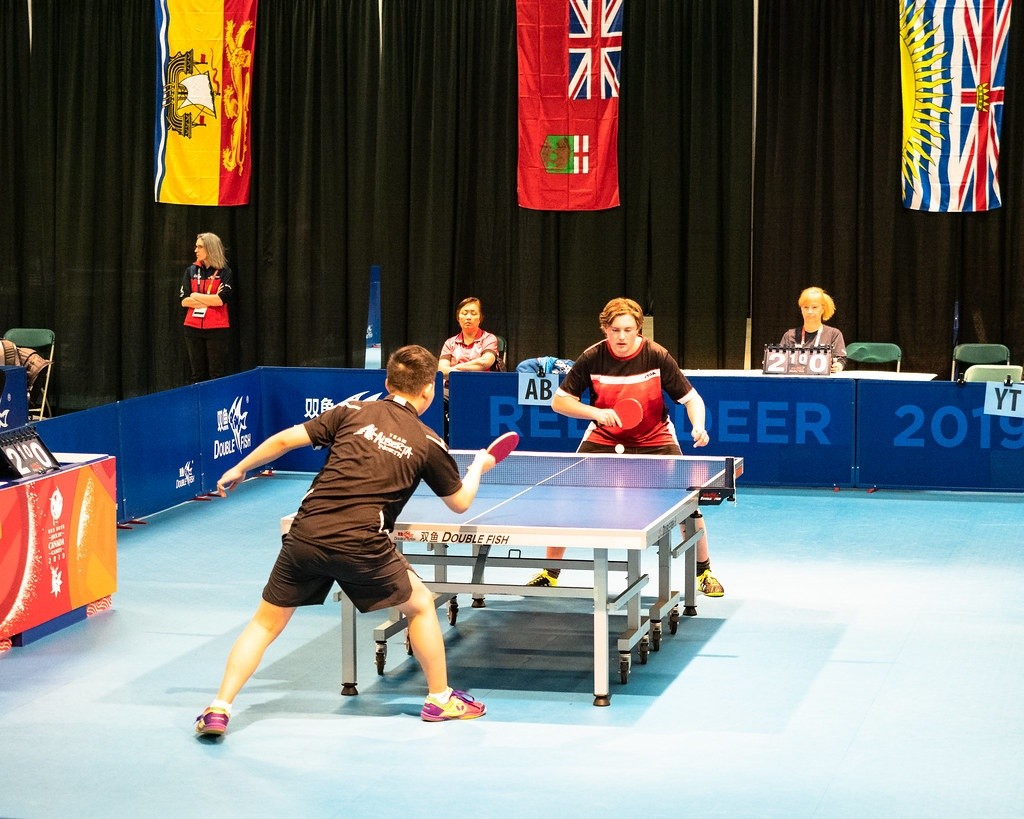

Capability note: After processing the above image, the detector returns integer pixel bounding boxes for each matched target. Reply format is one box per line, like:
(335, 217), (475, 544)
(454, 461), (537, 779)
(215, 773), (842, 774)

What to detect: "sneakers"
(525, 569), (557, 587)
(421, 687), (487, 721)
(697, 569), (725, 597)
(193, 706), (230, 735)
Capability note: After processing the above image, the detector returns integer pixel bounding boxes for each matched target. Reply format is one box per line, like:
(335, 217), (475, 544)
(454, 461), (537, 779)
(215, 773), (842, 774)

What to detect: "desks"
(680, 369), (939, 382)
(0, 452), (117, 651)
(280, 449), (744, 707)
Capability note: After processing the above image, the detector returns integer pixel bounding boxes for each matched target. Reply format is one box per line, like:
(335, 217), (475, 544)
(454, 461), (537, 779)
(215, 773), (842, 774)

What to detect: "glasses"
(193, 245), (205, 249)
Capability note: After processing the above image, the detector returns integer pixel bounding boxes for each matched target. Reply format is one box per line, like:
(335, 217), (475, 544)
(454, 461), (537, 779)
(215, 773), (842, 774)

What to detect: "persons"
(526, 297), (725, 597)
(180, 232), (232, 383)
(437, 298), (499, 403)
(193, 345), (495, 744)
(778, 286), (846, 376)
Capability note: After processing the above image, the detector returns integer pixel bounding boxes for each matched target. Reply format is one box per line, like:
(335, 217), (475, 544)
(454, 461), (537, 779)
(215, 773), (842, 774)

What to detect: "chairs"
(497, 335), (506, 367)
(845, 342), (902, 372)
(951, 343), (1023, 383)
(3, 328), (56, 422)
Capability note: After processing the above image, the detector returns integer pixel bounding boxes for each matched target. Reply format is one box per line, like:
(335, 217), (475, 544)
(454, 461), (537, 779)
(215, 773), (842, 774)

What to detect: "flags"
(155, 0), (258, 209)
(516, 0), (623, 211)
(898, 0), (1012, 212)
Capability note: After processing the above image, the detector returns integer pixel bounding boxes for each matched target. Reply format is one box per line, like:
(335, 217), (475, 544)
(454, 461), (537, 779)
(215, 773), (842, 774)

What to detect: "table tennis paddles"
(596, 397), (643, 430)
(466, 431), (520, 474)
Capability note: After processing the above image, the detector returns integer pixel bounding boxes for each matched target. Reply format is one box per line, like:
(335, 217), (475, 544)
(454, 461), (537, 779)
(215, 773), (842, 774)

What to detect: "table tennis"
(615, 444), (625, 454)
